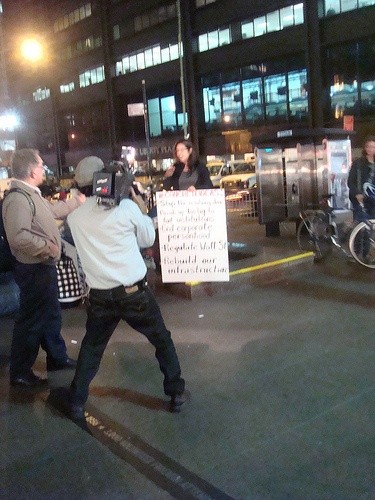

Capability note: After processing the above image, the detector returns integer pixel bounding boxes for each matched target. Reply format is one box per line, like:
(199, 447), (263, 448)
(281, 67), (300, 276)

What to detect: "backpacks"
(0, 188), (36, 272)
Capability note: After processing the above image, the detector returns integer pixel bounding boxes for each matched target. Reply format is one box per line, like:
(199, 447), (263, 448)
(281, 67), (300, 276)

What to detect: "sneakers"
(69, 404), (85, 420)
(170, 390), (190, 412)
(349, 253), (358, 263)
(363, 256), (370, 264)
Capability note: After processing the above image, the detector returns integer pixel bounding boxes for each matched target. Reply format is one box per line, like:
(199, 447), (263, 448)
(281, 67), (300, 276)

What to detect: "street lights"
(18, 37), (65, 181)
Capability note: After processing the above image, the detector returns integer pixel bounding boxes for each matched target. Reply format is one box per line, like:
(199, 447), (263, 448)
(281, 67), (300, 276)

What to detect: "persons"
(347, 136), (375, 264)
(163, 139), (213, 191)
(53, 156), (191, 419)
(1, 149), (87, 386)
(0, 177), (156, 315)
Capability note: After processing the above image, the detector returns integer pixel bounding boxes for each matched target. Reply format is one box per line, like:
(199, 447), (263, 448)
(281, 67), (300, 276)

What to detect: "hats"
(74, 155), (105, 187)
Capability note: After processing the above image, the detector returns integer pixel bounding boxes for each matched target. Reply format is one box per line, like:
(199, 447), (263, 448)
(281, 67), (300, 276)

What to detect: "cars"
(206, 163), (226, 187)
(219, 160), (255, 191)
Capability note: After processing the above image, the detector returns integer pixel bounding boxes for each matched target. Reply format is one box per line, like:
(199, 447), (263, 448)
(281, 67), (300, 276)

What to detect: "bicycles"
(297, 193), (375, 270)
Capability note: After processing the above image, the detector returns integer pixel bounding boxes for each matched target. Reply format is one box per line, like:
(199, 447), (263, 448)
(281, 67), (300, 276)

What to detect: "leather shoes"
(47, 357), (77, 372)
(10, 369), (48, 388)
